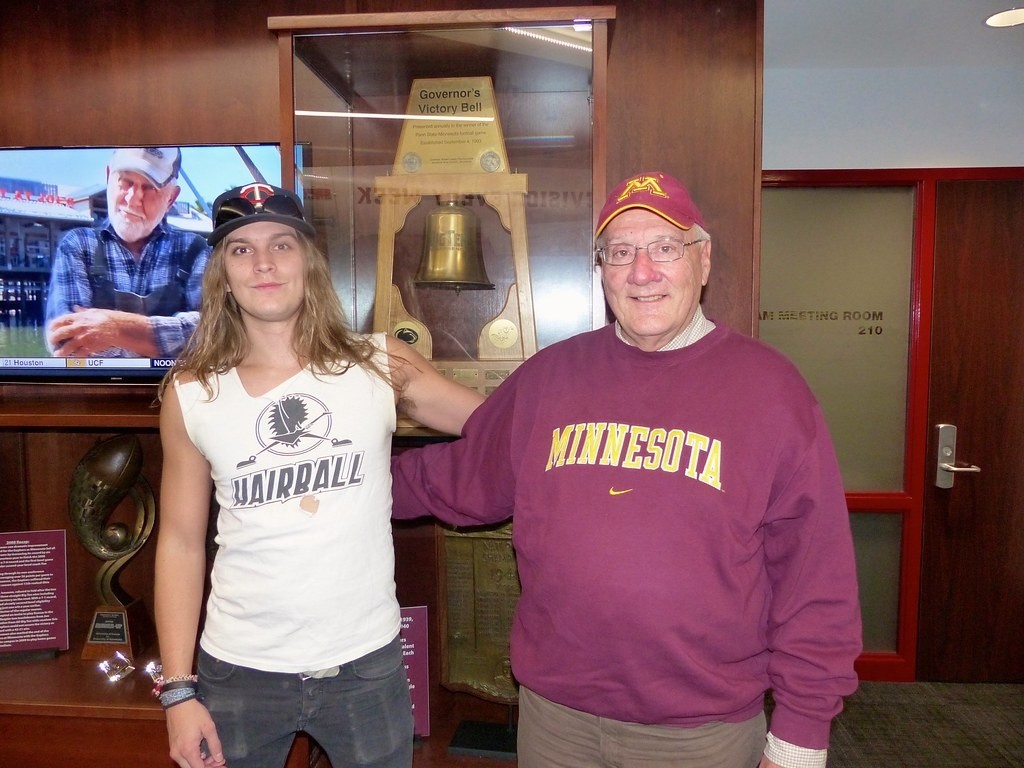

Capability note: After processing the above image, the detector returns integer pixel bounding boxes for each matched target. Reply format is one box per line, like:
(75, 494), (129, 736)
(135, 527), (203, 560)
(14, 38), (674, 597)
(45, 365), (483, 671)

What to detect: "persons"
(151, 179), (488, 768)
(44, 147), (219, 359)
(392, 172), (869, 768)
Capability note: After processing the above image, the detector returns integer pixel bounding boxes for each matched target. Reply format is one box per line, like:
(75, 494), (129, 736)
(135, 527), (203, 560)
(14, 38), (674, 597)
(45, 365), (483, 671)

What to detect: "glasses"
(214, 195), (304, 228)
(596, 238), (702, 266)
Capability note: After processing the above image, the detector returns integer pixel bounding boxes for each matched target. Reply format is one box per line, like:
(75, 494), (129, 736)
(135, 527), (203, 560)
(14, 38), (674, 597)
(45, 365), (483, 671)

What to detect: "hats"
(207, 182), (316, 246)
(594, 170), (709, 240)
(111, 147), (182, 191)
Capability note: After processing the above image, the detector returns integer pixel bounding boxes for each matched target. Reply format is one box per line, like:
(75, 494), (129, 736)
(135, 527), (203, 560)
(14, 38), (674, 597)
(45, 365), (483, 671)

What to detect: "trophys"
(67, 431), (157, 663)
(433, 518), (523, 762)
(371, 173), (540, 401)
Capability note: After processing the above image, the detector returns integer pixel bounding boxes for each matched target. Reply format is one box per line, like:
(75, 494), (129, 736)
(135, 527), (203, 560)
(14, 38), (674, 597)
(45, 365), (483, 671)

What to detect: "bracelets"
(153, 673), (197, 711)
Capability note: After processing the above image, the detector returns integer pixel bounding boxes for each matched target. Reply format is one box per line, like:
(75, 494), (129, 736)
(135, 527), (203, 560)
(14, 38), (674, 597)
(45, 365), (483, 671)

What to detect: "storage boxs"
(266, 4), (621, 438)
